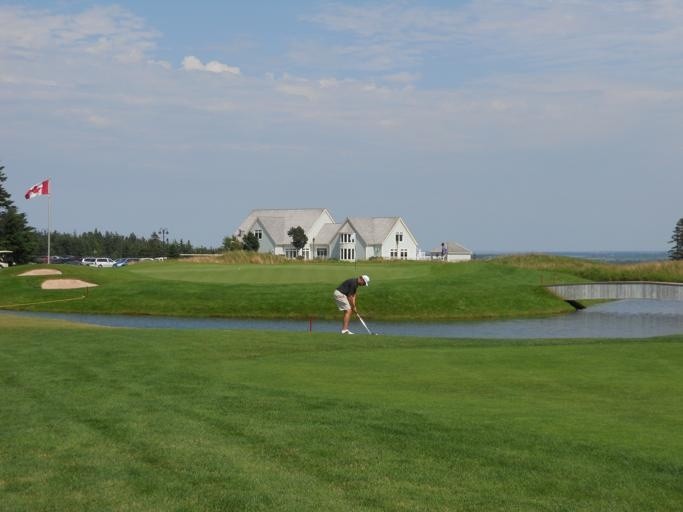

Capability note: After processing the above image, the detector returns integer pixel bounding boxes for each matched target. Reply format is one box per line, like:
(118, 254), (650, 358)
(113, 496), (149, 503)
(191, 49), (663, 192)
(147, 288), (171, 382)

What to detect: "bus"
(0, 251), (16, 269)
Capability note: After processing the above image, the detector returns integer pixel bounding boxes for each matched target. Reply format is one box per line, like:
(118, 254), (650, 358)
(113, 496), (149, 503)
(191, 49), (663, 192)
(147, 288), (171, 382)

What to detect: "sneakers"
(342, 329), (355, 335)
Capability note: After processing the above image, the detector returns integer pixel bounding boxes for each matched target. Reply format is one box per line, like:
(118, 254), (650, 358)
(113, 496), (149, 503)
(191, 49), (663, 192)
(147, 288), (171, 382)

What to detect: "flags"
(24, 177), (50, 200)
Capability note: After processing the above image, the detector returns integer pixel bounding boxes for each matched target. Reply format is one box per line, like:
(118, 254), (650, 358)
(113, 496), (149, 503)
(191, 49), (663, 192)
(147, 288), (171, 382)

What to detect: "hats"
(362, 275), (369, 287)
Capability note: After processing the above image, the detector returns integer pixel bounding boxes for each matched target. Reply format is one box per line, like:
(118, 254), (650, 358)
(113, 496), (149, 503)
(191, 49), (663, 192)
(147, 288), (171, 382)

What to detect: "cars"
(32, 255), (168, 269)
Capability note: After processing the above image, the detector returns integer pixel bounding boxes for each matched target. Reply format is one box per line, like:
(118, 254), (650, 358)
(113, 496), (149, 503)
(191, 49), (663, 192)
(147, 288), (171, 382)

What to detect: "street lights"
(158, 227), (169, 243)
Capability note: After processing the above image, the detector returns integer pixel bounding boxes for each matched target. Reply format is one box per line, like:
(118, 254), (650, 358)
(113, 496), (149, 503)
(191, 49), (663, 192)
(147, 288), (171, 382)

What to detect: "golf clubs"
(353, 307), (378, 335)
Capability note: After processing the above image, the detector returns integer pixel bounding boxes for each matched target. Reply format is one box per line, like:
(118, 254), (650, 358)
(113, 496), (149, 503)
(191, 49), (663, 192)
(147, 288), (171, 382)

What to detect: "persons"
(333, 274), (370, 334)
(441, 242), (448, 261)
